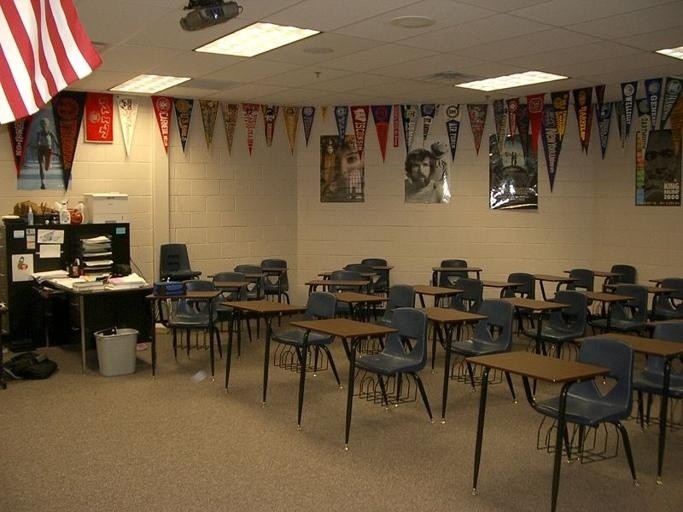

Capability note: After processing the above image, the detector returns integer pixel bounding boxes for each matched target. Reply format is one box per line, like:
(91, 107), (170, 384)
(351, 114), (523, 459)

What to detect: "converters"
(35, 353), (47, 362)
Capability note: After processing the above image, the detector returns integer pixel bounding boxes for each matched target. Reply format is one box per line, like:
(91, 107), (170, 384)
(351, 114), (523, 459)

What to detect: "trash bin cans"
(94, 328), (139, 376)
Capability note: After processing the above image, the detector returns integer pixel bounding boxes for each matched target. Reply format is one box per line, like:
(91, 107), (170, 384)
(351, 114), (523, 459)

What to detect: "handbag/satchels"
(5, 353), (57, 379)
(153, 282), (187, 295)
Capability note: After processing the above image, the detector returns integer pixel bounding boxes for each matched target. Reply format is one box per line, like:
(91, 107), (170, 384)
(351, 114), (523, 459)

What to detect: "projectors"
(180, 2), (239, 31)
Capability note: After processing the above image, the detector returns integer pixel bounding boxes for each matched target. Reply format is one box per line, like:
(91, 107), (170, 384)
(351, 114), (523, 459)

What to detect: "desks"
(215, 293), (309, 412)
(533, 273), (579, 305)
(287, 316), (397, 451)
(644, 285), (681, 316)
(303, 265), (395, 322)
(602, 331), (683, 489)
(480, 280), (525, 300)
(649, 277), (665, 287)
(463, 349), (610, 511)
(409, 282), (464, 351)
(420, 304), (489, 426)
(31, 267), (154, 379)
(488, 296), (572, 405)
(562, 269), (628, 318)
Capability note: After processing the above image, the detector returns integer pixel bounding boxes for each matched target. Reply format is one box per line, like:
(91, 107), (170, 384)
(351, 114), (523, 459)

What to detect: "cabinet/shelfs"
(3, 220), (132, 353)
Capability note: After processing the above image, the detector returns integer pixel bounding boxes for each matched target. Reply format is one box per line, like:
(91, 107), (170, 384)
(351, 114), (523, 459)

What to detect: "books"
(30, 235), (151, 292)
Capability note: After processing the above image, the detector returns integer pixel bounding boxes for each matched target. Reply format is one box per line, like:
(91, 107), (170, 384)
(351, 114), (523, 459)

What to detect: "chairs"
(534, 336), (643, 488)
(611, 319), (683, 489)
(154, 258), (292, 378)
(440, 294), (515, 400)
(155, 244), (204, 310)
(519, 290), (590, 360)
(631, 274), (683, 341)
(273, 290), (344, 390)
(431, 274), (485, 348)
(602, 264), (639, 317)
(498, 272), (536, 336)
(347, 306), (435, 424)
(440, 259), (469, 290)
(587, 282), (650, 337)
(328, 255), (390, 320)
(531, 265), (596, 329)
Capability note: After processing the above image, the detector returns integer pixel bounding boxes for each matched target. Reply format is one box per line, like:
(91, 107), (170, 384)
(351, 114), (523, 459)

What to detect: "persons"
(33, 116), (62, 189)
(404, 136), (445, 204)
(507, 149), (520, 167)
(643, 129), (681, 205)
(320, 136), (365, 202)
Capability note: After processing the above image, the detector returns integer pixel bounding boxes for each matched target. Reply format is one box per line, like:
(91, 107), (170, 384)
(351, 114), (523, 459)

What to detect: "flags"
(0, 0), (102, 125)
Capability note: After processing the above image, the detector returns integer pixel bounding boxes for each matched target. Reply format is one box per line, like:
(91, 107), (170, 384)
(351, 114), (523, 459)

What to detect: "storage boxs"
(80, 193), (128, 225)
